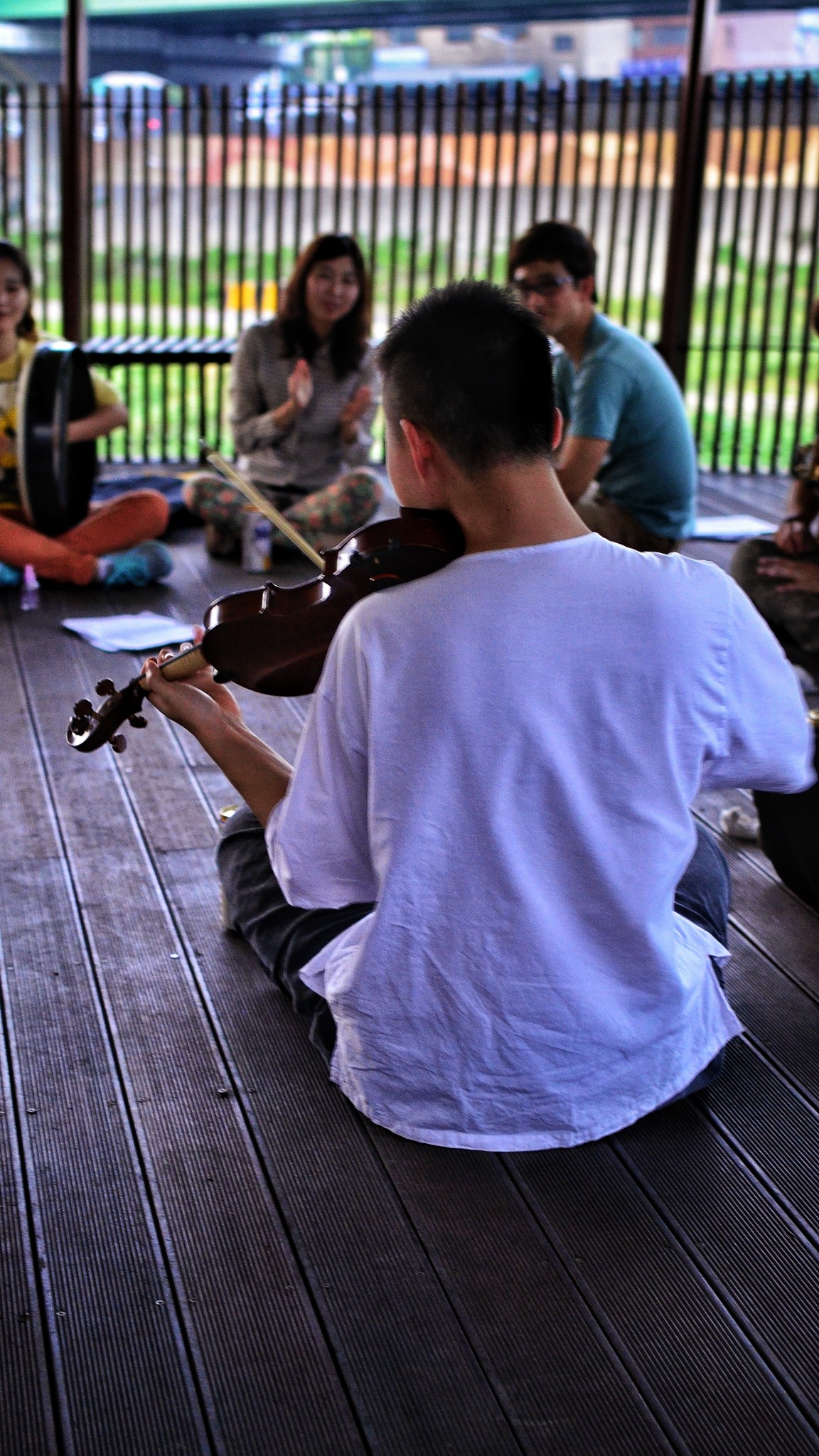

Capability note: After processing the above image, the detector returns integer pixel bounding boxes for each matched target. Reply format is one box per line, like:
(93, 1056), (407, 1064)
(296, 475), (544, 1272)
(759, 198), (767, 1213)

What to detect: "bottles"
(243, 501), (274, 572)
(21, 563), (41, 610)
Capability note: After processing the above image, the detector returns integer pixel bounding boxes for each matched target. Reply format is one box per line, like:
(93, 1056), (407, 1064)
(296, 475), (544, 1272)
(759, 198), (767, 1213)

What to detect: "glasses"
(510, 274), (574, 300)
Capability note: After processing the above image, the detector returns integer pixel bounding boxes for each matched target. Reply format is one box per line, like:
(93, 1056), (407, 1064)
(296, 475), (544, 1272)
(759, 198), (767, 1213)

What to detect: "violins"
(66, 505), (471, 753)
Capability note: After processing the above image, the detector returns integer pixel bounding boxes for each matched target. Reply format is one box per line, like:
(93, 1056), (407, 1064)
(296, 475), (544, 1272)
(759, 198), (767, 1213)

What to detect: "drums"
(18, 341), (95, 538)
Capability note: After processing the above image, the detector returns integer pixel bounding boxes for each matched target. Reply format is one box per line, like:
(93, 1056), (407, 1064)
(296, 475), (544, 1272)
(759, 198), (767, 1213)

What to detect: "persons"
(135, 283), (819, 1152)
(0, 244), (171, 591)
(504, 222), (697, 554)
(181, 233), (382, 562)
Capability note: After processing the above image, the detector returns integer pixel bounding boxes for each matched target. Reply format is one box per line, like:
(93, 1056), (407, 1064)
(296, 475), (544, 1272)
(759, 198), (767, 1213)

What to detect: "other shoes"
(0, 564), (23, 585)
(103, 540), (174, 589)
